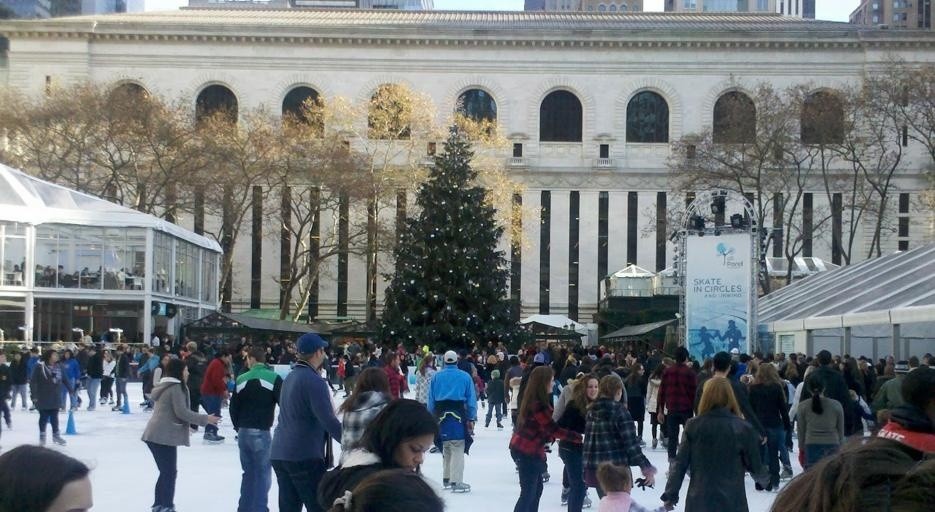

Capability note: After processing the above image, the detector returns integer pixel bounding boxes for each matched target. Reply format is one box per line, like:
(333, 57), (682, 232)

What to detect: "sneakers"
(9, 397), (153, 415)
(451, 482), (470, 489)
(443, 480), (449, 486)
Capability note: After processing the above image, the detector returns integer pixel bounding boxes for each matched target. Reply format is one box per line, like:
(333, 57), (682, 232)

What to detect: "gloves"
(635, 478), (653, 490)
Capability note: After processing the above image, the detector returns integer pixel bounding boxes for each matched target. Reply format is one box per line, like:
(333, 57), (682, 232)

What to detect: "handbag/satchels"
(316, 466), (356, 510)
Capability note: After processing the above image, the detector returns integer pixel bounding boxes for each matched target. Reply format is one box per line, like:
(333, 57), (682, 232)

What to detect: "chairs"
(11, 272), (22, 285)
(131, 277), (142, 289)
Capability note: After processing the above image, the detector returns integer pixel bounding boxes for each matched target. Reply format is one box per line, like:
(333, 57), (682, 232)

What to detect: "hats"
(534, 354), (544, 363)
(296, 332), (330, 353)
(491, 370), (501, 379)
(444, 349), (458, 364)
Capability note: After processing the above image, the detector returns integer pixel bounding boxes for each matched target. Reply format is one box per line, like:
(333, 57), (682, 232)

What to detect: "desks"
(81, 275), (95, 288)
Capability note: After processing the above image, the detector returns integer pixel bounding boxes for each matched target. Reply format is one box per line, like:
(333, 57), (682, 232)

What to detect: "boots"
(562, 488), (571, 503)
(637, 430), (668, 448)
(582, 490), (592, 506)
(53, 430), (65, 443)
(780, 464), (792, 478)
(477, 399), (508, 429)
(204, 424), (225, 441)
(39, 431), (47, 445)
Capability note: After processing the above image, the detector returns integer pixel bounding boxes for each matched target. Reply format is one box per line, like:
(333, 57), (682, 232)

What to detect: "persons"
(689, 326), (722, 360)
(3, 256), (186, 296)
(721, 318), (745, 353)
(1, 333), (934, 512)
